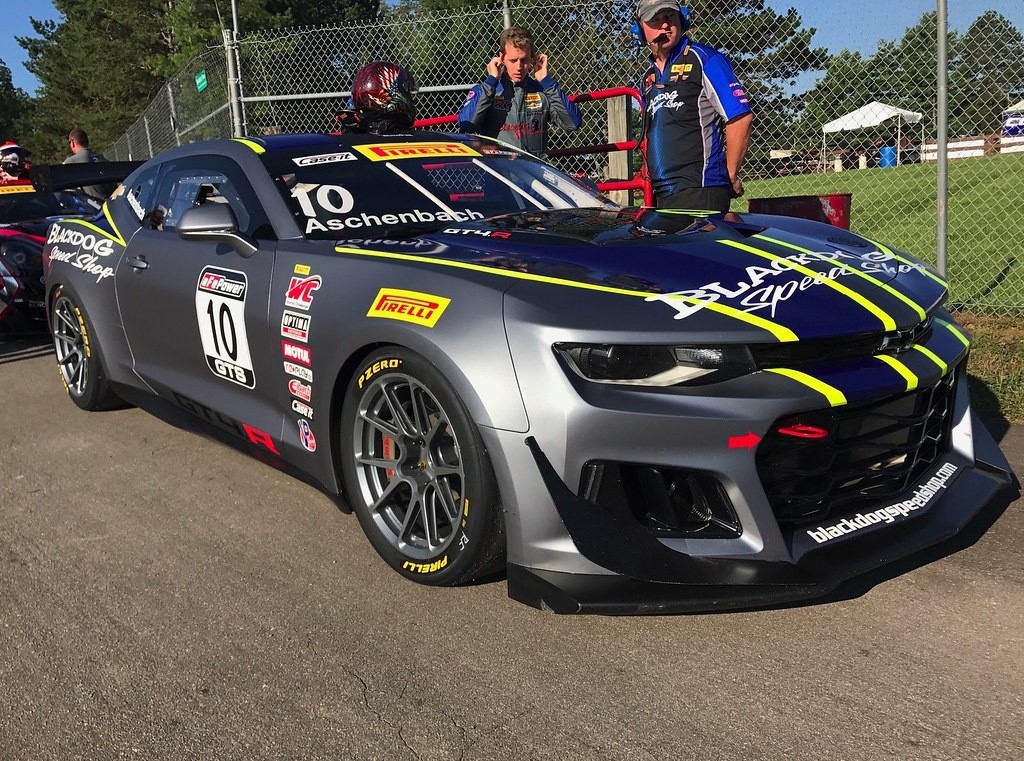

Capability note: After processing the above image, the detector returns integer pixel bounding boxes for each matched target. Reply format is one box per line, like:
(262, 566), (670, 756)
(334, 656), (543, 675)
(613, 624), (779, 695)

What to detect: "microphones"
(640, 33), (667, 47)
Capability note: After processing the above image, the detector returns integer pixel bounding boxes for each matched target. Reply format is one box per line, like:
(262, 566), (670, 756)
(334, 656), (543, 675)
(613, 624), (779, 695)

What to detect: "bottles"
(859, 154), (866, 169)
(835, 156), (842, 172)
(348, 98), (354, 110)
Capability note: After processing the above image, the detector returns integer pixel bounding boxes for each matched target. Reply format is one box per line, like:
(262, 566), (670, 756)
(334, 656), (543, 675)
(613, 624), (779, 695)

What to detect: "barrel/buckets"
(880, 146), (896, 168)
(748, 193), (852, 230)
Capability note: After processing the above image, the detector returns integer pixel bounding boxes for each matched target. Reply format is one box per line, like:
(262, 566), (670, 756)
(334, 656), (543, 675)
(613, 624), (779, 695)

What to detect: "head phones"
(630, 6), (692, 48)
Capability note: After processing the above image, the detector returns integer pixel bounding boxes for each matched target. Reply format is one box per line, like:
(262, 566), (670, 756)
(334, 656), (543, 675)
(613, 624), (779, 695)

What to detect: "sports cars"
(27, 128), (1018, 613)
(0, 180), (104, 339)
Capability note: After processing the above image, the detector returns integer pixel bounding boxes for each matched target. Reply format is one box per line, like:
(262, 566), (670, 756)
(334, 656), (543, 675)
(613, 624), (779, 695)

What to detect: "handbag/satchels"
(88, 150), (118, 200)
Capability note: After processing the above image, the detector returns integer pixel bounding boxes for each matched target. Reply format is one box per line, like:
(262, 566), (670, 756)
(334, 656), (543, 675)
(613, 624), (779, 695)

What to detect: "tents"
(1001, 100), (1024, 123)
(813, 101), (924, 173)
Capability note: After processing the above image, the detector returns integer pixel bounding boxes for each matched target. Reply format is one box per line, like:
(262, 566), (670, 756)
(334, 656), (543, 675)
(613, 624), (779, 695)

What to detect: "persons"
(0, 142), (34, 181)
(336, 60), (417, 134)
(59, 130), (119, 202)
(636, 0), (754, 212)
(458, 26), (582, 208)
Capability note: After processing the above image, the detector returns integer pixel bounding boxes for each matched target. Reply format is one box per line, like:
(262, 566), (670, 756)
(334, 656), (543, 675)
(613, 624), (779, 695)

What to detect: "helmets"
(351, 61), (419, 131)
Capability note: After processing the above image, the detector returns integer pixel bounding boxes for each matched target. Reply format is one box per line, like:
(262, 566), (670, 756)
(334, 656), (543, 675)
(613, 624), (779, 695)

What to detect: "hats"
(636, 0), (681, 21)
